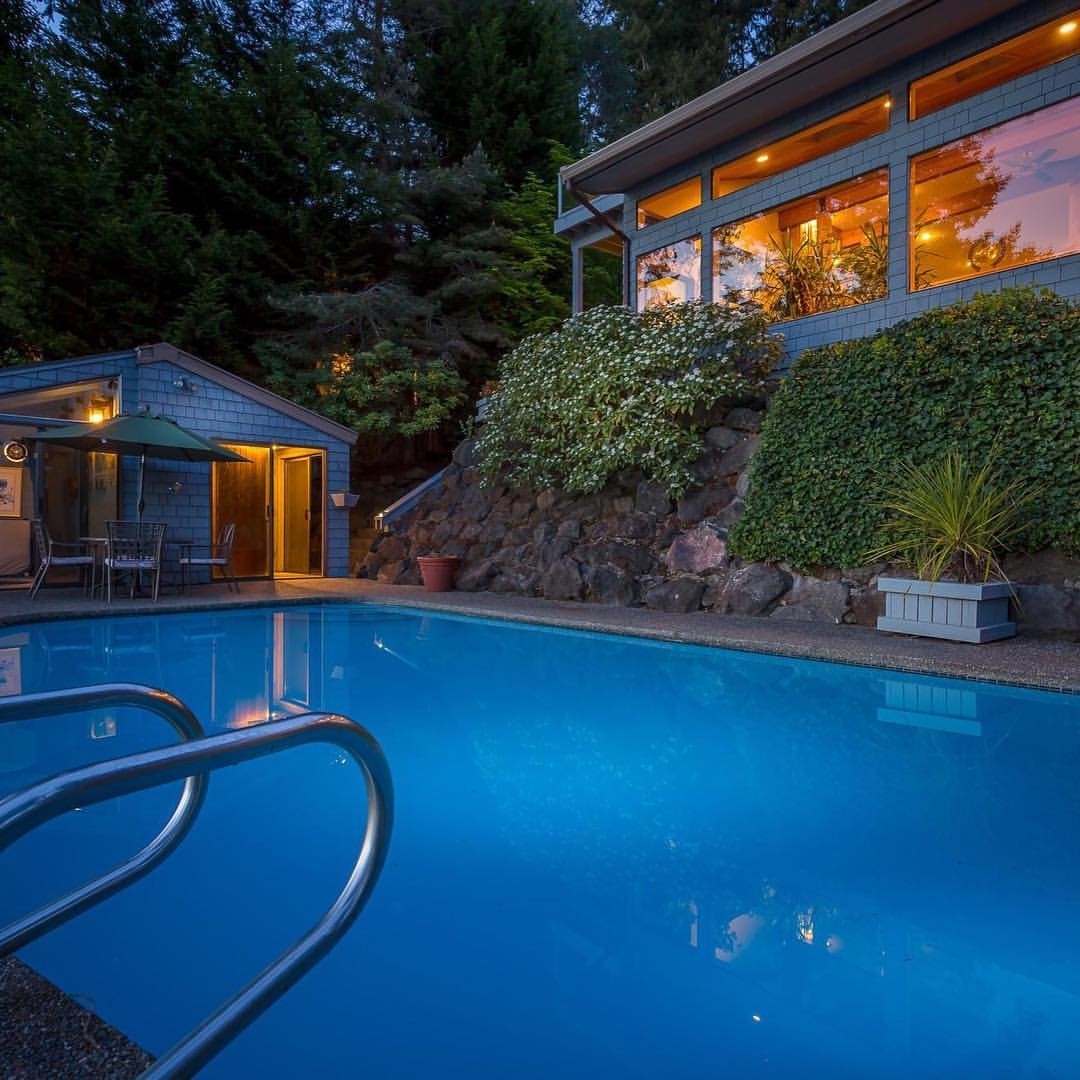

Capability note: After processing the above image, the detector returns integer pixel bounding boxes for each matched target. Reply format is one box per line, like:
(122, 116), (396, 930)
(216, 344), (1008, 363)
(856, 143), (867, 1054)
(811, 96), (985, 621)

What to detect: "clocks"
(967, 231), (1006, 269)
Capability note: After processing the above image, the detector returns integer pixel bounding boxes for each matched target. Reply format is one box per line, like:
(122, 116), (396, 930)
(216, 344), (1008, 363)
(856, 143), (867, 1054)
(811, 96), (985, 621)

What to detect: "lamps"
(174, 374), (199, 395)
(88, 401), (106, 422)
(168, 481), (184, 494)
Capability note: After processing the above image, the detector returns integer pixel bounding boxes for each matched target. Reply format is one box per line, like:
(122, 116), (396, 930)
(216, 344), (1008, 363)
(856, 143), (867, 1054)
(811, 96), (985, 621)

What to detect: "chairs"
(27, 512), (241, 604)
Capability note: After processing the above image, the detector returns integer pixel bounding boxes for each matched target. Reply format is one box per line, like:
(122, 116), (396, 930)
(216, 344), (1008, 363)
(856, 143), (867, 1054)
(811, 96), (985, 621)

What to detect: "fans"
(1001, 148), (1061, 181)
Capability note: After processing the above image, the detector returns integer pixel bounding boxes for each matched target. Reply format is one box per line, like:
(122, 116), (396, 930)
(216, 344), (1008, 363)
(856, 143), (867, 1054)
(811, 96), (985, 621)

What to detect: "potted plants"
(417, 549), (459, 591)
(328, 489), (360, 509)
(857, 436), (1045, 644)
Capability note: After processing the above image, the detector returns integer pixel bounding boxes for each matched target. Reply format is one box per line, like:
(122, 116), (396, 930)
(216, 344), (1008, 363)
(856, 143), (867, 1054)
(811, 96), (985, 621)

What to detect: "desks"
(80, 536), (196, 599)
